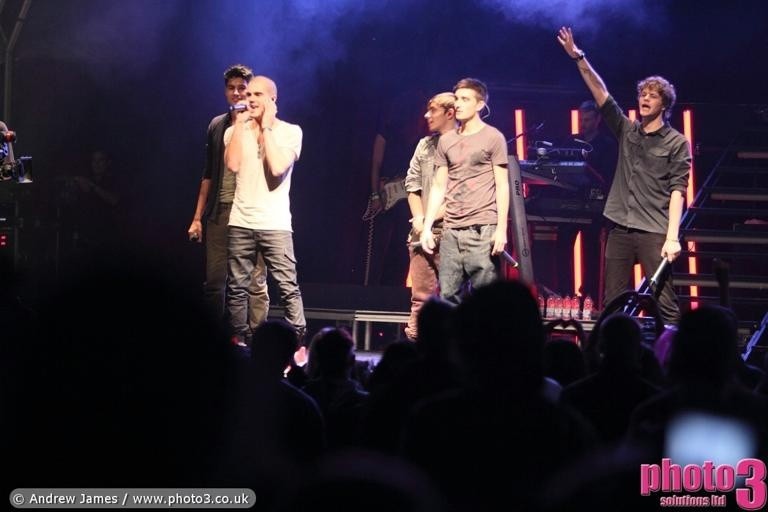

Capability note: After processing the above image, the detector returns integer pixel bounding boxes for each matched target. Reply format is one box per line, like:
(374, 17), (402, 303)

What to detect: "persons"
(370, 119), (405, 283)
(190, 64), (270, 331)
(420, 77), (510, 308)
(557, 101), (616, 305)
(555, 24), (691, 332)
(403, 91), (458, 343)
(0, 248), (767, 512)
(73, 149), (122, 219)
(223, 75), (306, 345)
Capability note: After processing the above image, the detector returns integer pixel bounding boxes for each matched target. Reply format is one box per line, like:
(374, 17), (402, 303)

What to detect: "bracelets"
(371, 191), (379, 200)
(573, 53), (585, 63)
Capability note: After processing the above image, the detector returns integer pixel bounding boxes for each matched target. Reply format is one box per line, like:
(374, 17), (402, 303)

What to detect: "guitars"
(363, 176), (408, 221)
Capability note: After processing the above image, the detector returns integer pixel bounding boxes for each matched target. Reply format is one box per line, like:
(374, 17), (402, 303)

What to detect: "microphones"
(229, 103), (248, 113)
(489, 240), (523, 271)
(412, 237), (439, 250)
(193, 232), (204, 246)
